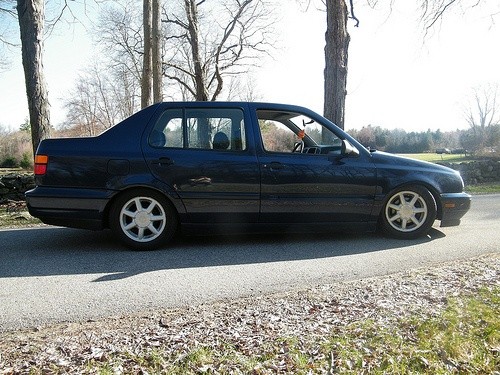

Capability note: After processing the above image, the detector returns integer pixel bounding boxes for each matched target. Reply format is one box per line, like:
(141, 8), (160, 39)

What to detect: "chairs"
(149, 130), (167, 148)
(213, 132), (231, 150)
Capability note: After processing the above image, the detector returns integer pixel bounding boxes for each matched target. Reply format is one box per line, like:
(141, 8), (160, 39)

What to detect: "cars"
(435, 147), (469, 154)
(25, 100), (472, 251)
